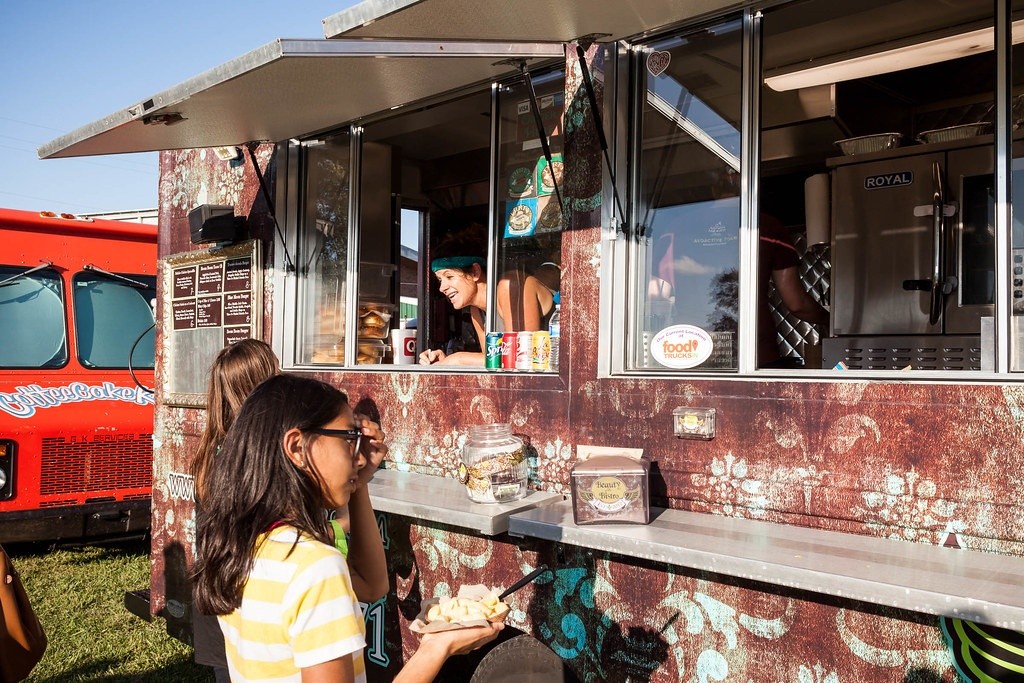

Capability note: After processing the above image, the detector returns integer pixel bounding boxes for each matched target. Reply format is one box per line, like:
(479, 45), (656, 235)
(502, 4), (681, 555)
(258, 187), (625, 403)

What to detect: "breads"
(309, 302), (389, 365)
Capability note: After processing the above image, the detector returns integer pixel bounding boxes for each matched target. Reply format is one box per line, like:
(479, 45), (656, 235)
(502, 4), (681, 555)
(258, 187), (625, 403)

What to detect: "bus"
(0, 207), (161, 543)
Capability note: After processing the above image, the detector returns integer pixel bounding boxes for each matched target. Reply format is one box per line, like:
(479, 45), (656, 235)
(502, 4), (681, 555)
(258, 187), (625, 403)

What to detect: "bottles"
(463, 424), (527, 504)
(549, 304), (561, 372)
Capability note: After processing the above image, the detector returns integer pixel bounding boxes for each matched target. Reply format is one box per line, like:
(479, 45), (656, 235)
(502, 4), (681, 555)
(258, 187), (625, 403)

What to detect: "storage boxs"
(303, 261), (399, 365)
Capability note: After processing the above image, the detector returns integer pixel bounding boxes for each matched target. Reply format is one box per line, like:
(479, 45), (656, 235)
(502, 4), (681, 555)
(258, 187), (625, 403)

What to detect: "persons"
(418, 231), (562, 367)
(194, 375), (505, 683)
(192, 339), (281, 683)
(709, 162), (830, 364)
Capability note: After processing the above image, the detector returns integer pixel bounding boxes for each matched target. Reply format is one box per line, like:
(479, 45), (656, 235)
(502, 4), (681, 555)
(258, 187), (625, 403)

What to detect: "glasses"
(296, 428), (365, 460)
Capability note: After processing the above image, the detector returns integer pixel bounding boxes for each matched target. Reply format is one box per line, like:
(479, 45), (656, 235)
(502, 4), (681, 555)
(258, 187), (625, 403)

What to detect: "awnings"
(35, 0), (754, 271)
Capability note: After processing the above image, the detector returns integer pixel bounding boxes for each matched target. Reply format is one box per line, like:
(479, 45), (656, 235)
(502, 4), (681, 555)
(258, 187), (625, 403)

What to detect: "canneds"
(485, 330), (550, 371)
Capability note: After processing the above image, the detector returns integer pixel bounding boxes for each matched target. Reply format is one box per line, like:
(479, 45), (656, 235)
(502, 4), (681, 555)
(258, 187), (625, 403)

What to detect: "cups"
(391, 329), (417, 365)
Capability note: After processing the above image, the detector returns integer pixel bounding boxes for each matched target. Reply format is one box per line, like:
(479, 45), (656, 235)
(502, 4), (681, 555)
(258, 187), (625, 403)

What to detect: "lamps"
(761, 12), (1024, 92)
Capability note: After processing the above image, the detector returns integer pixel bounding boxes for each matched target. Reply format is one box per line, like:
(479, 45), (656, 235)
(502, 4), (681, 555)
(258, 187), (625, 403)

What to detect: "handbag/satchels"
(0, 544), (48, 683)
